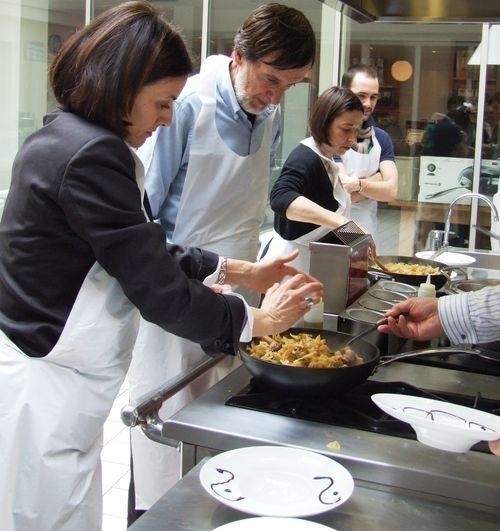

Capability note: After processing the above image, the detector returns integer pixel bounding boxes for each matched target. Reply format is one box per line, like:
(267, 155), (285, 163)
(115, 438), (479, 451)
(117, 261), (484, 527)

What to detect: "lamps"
(390, 45), (412, 82)
(465, 23), (500, 68)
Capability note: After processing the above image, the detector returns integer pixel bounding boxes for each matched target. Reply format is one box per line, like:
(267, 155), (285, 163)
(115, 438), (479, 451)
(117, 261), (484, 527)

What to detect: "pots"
(367, 245), (450, 291)
(235, 327), (499, 395)
(424, 166), (500, 200)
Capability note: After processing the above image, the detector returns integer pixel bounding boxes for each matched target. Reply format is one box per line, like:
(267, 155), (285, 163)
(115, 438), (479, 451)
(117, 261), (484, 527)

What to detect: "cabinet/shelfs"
(449, 47), (499, 120)
(372, 82), (405, 120)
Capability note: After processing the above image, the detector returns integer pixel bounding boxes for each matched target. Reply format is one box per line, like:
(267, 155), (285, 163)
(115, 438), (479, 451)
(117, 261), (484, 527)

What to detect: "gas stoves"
(222, 377), (500, 458)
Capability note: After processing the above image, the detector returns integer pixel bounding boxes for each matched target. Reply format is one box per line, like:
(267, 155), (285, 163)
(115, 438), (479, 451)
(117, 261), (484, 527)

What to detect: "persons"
(0, 1), (325, 531)
(420, 92), (499, 158)
(257, 85), (377, 330)
(335, 65), (399, 258)
(378, 282), (499, 457)
(126, 3), (316, 527)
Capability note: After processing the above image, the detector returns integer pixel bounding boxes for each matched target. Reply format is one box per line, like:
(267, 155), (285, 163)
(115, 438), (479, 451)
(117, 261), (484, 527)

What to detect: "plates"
(369, 392), (500, 451)
(214, 517), (338, 529)
(198, 445), (356, 518)
(413, 250), (476, 266)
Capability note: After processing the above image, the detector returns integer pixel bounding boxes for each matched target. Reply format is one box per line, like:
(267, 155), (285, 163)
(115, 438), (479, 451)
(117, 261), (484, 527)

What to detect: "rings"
(304, 293), (314, 308)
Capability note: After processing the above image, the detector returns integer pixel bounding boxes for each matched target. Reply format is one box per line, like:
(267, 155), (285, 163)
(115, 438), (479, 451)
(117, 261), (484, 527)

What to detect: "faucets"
(443, 192), (500, 248)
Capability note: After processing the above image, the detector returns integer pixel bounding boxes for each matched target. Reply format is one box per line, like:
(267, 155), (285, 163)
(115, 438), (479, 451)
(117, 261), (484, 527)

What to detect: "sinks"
(454, 250), (500, 272)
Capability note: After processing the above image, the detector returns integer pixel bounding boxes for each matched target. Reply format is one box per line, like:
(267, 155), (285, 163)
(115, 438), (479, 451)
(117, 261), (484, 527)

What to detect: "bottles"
(418, 273), (438, 300)
(302, 294), (326, 324)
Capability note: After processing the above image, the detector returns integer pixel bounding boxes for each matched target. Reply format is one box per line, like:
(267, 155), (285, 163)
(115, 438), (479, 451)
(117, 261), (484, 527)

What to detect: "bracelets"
(216, 256), (228, 286)
(355, 177), (364, 193)
